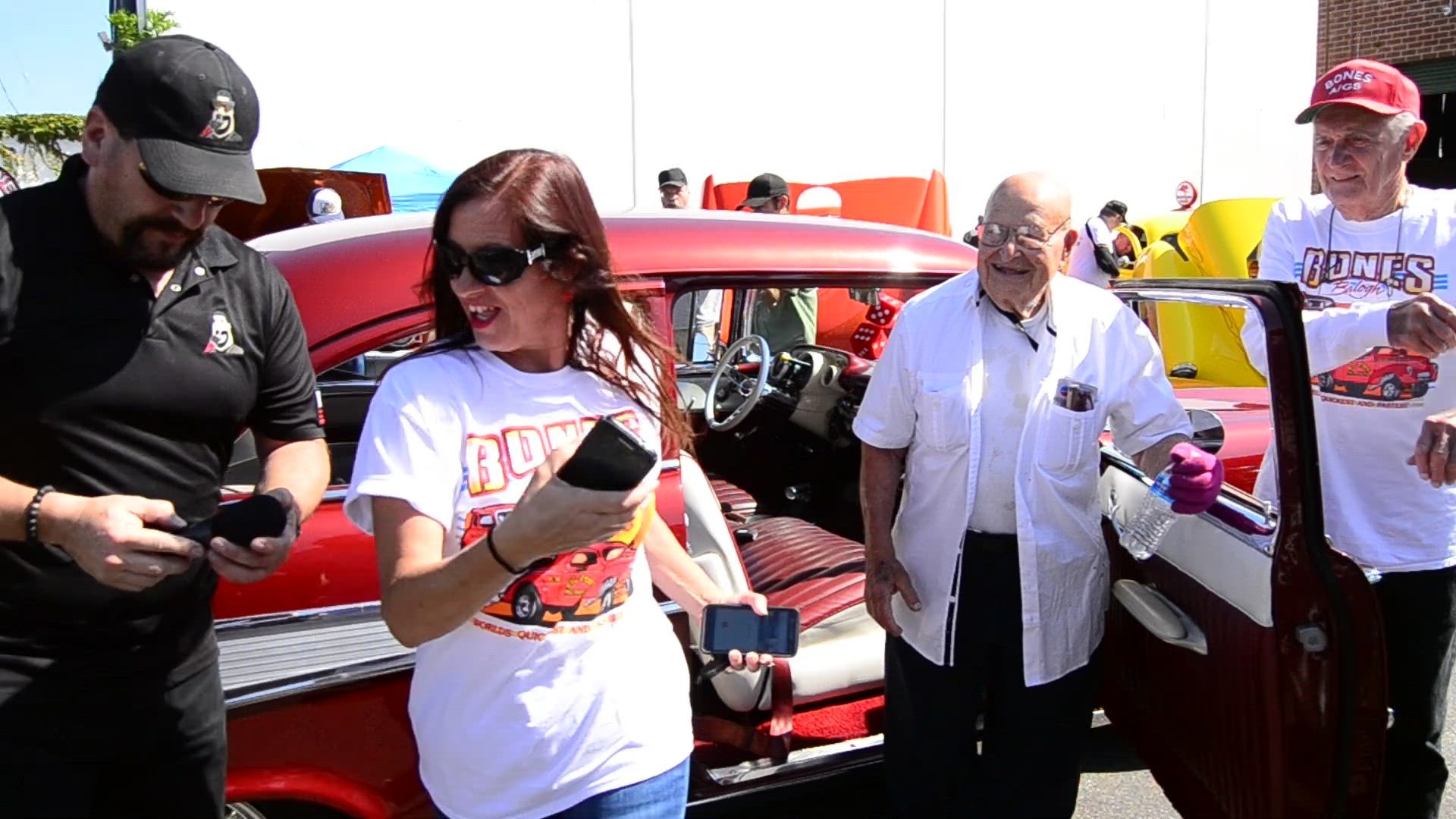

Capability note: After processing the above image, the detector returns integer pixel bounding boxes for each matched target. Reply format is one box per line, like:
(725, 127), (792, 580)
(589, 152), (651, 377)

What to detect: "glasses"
(975, 216), (1071, 251)
(433, 238), (549, 285)
(137, 160), (234, 206)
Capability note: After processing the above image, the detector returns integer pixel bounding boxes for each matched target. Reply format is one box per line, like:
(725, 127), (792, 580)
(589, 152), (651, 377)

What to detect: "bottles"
(1119, 461), (1179, 560)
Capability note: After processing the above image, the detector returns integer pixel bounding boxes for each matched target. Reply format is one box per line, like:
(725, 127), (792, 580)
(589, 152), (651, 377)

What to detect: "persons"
(304, 187), (346, 227)
(737, 174), (819, 359)
(852, 173), (1224, 819)
(0, 37), (332, 819)
(1241, 57), (1456, 819)
(1068, 200), (1142, 291)
(343, 148), (775, 819)
(658, 169), (717, 365)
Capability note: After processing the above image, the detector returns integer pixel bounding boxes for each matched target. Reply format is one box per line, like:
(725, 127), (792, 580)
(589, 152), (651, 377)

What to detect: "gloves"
(1166, 443), (1224, 515)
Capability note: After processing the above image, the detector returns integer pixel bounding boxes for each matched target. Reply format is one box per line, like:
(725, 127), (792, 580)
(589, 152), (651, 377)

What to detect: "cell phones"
(699, 602), (801, 658)
(557, 414), (658, 494)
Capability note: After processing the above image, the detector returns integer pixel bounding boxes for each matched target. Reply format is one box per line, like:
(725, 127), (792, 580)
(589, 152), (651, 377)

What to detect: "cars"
(1095, 384), (1277, 491)
(695, 168), (957, 363)
(200, 209), (1392, 819)
(1119, 197), (1279, 386)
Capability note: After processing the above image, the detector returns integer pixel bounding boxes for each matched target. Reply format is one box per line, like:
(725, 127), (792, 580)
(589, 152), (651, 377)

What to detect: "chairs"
(678, 446), (887, 705)
(707, 476), (760, 521)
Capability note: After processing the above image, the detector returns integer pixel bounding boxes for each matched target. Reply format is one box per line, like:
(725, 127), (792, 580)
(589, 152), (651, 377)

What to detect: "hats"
(1106, 200), (1128, 224)
(658, 168), (687, 187)
(104, 34), (267, 204)
(1296, 58), (1421, 124)
(736, 172), (789, 211)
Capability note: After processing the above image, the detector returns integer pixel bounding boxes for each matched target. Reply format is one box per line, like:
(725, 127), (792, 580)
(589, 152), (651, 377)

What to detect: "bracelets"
(487, 524), (530, 575)
(25, 484), (56, 547)
(294, 506), (301, 538)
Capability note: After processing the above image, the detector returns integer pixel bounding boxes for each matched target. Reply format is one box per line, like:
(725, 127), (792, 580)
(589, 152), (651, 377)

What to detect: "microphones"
(159, 493), (288, 557)
